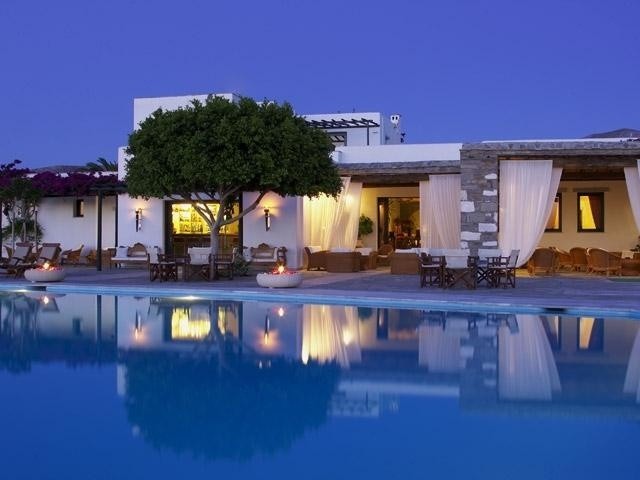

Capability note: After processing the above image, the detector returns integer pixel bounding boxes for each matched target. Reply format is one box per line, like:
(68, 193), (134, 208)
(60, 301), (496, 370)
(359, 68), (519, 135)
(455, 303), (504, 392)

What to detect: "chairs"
(0, 242), (115, 280)
(417, 311), (520, 335)
(111, 243), (287, 283)
(415, 248), (519, 290)
(304, 245), (420, 275)
(527, 245), (638, 278)
(388, 229), (421, 248)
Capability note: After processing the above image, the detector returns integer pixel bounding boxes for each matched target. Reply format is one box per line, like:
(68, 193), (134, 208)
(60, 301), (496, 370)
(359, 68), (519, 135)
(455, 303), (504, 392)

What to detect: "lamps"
(134, 311), (139, 340)
(263, 316), (271, 345)
(265, 209), (271, 231)
(136, 208), (143, 233)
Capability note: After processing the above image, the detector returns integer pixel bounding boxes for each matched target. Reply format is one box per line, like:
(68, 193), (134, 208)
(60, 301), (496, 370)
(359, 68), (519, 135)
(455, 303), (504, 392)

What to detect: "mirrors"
(376, 196), (420, 248)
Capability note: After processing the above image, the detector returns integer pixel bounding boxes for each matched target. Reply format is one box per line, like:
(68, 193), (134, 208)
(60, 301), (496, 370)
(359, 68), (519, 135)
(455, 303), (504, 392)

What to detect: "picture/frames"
(577, 317), (606, 353)
(542, 191), (562, 233)
(576, 192), (604, 231)
(545, 315), (561, 352)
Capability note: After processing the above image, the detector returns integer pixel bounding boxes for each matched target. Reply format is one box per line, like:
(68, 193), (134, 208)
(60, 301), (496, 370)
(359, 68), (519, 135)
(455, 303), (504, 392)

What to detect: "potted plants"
(356, 214), (373, 247)
